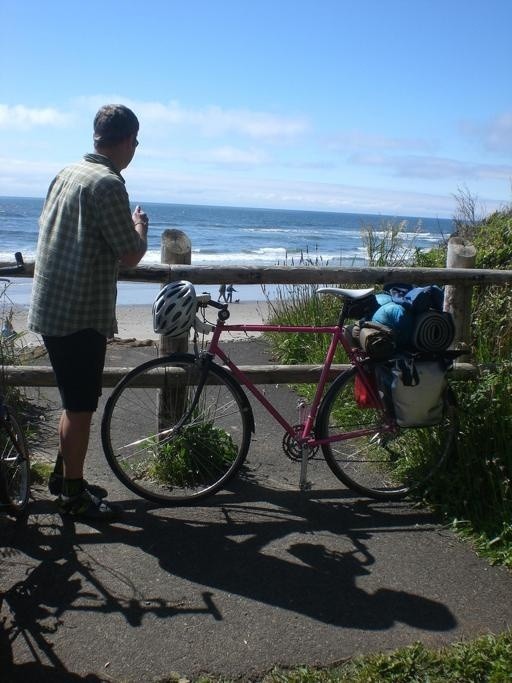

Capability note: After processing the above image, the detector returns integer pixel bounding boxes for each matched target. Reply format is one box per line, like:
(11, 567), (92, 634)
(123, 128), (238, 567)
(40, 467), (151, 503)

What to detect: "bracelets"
(134, 222), (147, 227)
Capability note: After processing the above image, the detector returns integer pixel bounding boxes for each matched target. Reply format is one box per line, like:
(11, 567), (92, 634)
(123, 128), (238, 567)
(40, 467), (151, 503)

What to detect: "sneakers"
(48, 473), (122, 520)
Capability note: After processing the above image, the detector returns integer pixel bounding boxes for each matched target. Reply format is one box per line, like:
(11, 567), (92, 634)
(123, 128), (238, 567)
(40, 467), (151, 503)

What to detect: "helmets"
(152, 280), (197, 337)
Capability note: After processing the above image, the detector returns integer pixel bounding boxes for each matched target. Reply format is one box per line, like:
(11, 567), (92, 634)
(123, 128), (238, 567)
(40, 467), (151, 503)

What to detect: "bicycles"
(0, 252), (31, 515)
(101, 279), (470, 505)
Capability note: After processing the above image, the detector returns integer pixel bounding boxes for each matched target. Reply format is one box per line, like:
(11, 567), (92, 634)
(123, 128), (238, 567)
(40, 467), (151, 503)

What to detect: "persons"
(218, 284), (237, 303)
(28, 105), (149, 513)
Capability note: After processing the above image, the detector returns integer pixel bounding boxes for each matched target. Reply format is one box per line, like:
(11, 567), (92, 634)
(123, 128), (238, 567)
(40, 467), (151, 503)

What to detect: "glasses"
(135, 140), (138, 146)
